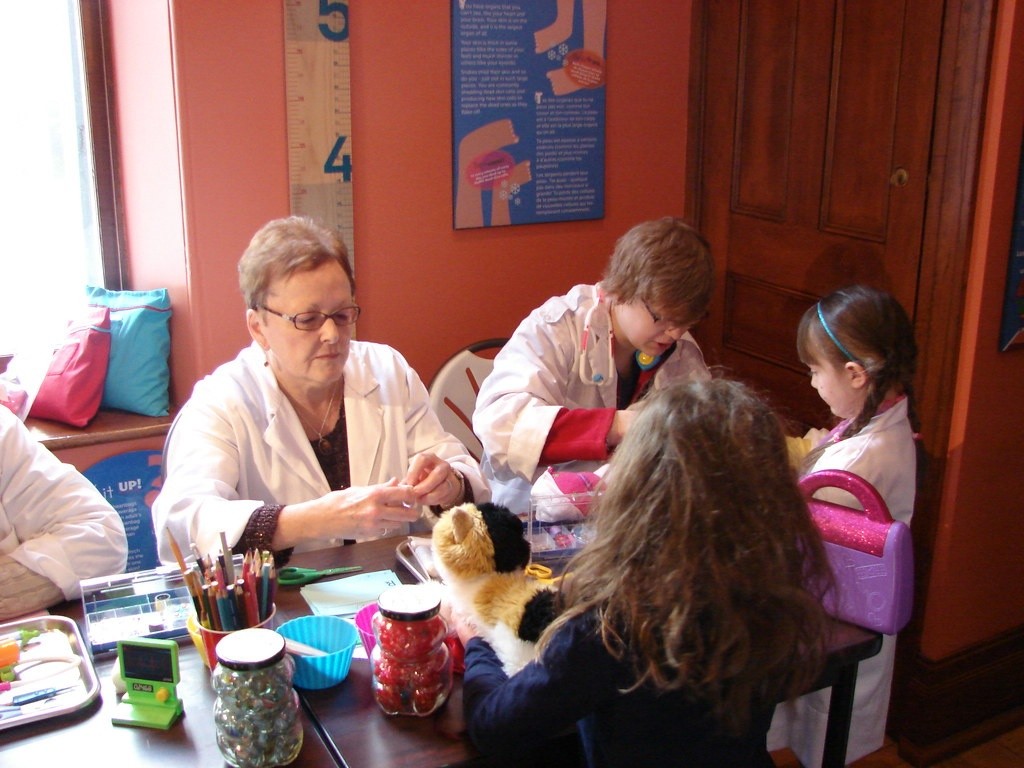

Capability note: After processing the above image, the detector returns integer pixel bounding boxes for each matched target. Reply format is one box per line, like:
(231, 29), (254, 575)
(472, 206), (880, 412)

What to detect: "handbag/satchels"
(796, 468), (913, 634)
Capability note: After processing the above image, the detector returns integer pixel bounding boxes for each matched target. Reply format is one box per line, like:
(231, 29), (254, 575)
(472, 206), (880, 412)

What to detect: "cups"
(196, 602), (275, 670)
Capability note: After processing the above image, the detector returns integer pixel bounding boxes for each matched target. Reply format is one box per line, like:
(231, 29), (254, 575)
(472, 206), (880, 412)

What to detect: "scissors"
(278, 566), (365, 587)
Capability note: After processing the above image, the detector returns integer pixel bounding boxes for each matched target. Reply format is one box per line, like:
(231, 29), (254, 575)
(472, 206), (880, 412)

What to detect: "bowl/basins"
(276, 615), (360, 688)
(187, 615), (208, 665)
(355, 602), (379, 657)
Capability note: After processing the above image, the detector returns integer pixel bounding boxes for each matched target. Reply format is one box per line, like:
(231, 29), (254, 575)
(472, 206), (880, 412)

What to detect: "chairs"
(425, 337), (510, 462)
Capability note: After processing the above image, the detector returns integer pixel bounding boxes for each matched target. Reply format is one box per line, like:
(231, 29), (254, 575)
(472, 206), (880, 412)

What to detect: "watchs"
(445, 467), (466, 508)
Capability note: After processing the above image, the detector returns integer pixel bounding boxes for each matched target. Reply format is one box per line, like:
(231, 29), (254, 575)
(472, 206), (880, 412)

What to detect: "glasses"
(641, 296), (695, 331)
(252, 295), (361, 331)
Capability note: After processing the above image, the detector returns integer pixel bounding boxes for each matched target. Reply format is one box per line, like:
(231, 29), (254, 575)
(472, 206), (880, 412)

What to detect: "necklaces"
(294, 384), (334, 456)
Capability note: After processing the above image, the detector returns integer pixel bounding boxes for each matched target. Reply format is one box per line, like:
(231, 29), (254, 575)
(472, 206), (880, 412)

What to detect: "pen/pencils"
(162, 524), (278, 634)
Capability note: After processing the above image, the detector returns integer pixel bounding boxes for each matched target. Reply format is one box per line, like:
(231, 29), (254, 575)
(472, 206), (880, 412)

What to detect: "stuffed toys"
(530, 459), (612, 522)
(429, 503), (567, 675)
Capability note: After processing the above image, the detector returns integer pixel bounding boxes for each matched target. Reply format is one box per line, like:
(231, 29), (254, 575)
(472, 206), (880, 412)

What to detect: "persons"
(765, 286), (935, 768)
(0, 403), (127, 620)
(472, 217), (715, 515)
(455, 372), (833, 768)
(150, 216), (491, 571)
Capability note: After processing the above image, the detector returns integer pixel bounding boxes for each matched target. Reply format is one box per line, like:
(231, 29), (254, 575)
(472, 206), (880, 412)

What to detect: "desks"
(266, 529), (883, 768)
(0, 590), (338, 768)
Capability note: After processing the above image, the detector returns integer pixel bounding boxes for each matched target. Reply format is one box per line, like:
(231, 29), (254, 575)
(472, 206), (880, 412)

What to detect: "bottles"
(212, 628), (303, 768)
(370, 585), (453, 717)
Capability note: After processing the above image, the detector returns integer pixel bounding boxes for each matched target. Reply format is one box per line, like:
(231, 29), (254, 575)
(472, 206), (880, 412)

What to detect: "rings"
(446, 479), (452, 488)
(382, 528), (388, 536)
